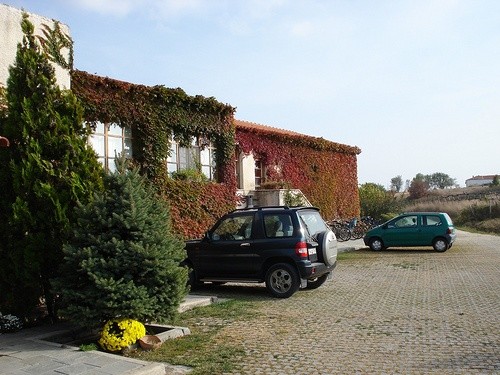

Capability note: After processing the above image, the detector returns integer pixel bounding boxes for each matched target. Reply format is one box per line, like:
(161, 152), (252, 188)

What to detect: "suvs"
(181, 203), (341, 299)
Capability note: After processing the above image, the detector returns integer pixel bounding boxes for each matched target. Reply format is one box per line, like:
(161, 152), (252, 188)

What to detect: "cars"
(364, 211), (457, 252)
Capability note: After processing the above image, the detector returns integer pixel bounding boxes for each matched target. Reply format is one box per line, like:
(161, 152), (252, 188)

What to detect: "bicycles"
(327, 216), (381, 242)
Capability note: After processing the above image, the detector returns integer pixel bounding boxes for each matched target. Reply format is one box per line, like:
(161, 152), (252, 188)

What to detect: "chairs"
(274, 221), (283, 237)
(412, 218), (416, 226)
